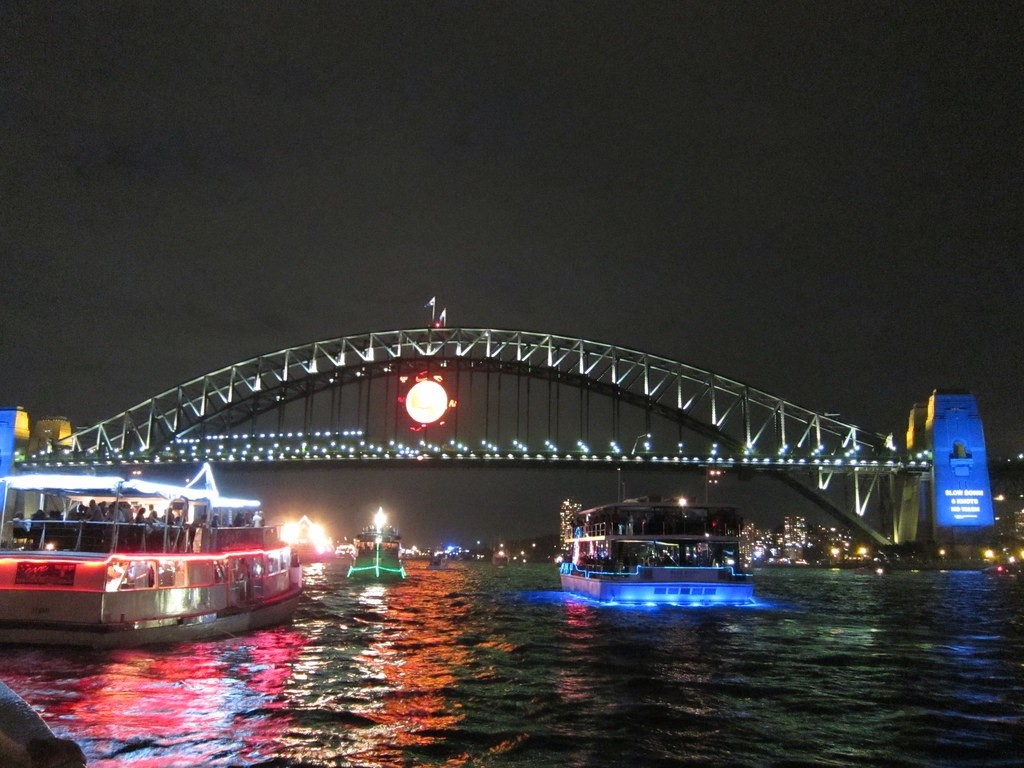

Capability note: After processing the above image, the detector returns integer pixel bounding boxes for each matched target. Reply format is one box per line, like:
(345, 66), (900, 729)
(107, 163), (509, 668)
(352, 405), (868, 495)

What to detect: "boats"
(0, 473), (307, 651)
(426, 552), (450, 570)
(559, 502), (756, 603)
(347, 532), (409, 582)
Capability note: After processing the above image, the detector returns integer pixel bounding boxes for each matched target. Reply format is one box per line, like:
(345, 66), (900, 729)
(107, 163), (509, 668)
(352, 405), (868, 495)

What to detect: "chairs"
(11, 528), (30, 549)
(32, 527), (194, 550)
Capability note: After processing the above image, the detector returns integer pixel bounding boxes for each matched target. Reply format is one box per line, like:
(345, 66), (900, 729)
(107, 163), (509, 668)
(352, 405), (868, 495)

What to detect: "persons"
(13, 496), (266, 559)
(356, 542), (399, 560)
(581, 550), (712, 572)
